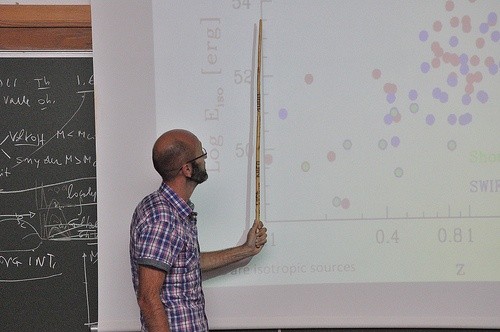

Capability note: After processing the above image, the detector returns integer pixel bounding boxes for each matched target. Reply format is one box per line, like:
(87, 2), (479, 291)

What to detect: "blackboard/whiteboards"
(0, 51), (98, 332)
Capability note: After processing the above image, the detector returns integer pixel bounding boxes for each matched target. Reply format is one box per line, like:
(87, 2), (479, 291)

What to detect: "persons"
(129, 129), (267, 332)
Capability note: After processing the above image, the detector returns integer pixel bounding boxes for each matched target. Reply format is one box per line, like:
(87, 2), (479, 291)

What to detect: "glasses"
(179, 146), (208, 174)
